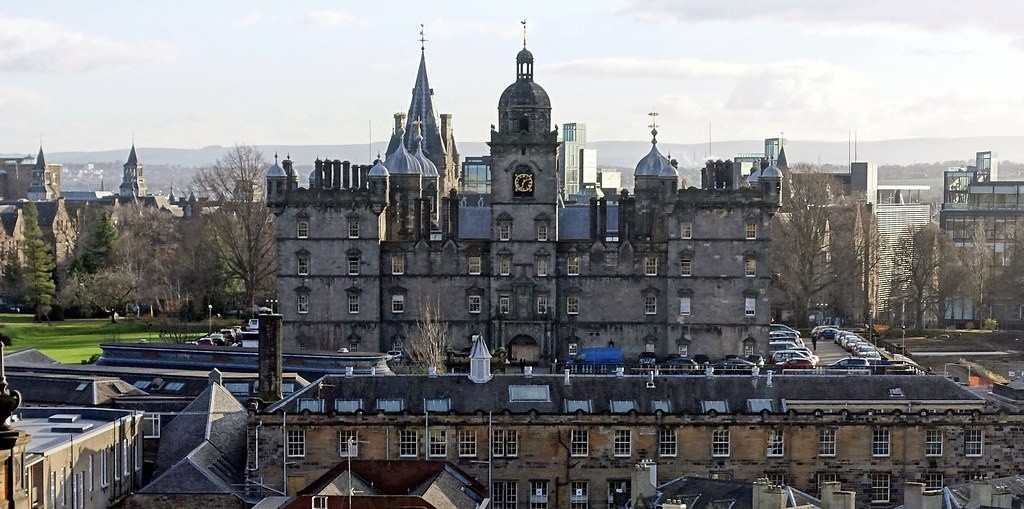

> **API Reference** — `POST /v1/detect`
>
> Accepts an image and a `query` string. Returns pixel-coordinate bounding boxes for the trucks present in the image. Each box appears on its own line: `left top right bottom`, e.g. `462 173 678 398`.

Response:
565 348 624 373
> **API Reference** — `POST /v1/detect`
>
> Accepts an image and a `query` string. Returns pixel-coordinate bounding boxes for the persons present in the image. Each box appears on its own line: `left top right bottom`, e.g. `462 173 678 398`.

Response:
705 365 714 375
812 334 817 351
520 357 526 373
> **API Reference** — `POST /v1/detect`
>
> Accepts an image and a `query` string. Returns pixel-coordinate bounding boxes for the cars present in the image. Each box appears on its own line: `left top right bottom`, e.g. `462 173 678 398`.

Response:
775 358 813 372
712 358 757 375
185 319 259 346
660 359 699 374
811 325 884 360
770 331 819 364
638 352 658 368
769 324 801 337
825 357 870 370
386 351 403 361
748 354 767 368
722 355 739 360
692 355 711 369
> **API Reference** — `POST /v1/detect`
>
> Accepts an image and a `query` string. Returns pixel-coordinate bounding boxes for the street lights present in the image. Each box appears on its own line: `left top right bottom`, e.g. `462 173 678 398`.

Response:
266 300 278 311
209 304 212 334
816 303 828 325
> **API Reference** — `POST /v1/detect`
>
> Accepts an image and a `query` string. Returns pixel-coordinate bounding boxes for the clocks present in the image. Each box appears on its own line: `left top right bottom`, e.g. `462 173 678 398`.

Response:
513 172 533 191
